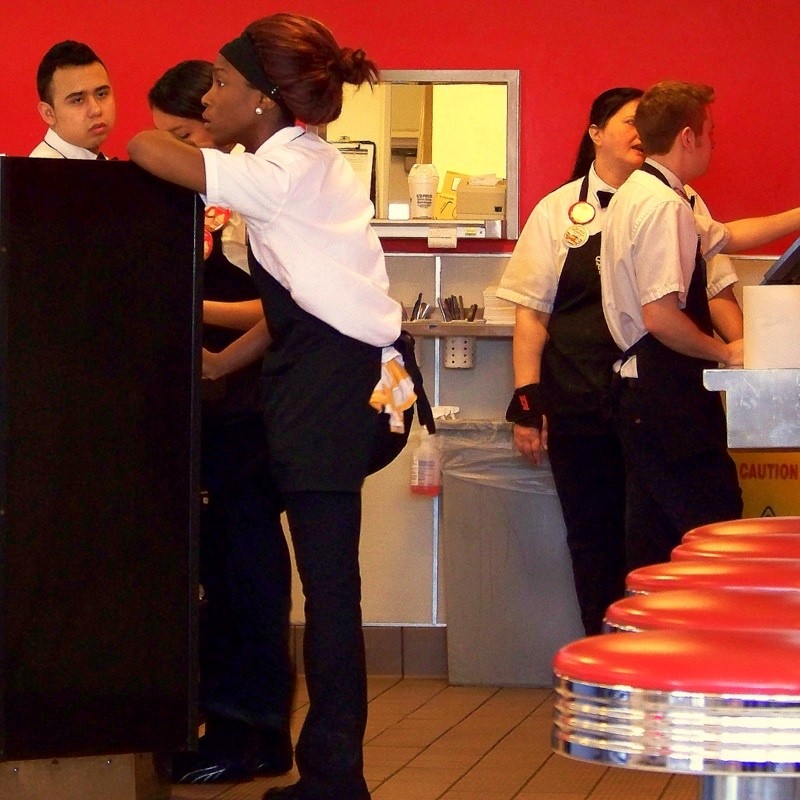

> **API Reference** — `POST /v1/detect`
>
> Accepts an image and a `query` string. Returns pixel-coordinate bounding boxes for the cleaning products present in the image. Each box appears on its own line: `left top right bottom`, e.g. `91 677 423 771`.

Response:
408 406 460 497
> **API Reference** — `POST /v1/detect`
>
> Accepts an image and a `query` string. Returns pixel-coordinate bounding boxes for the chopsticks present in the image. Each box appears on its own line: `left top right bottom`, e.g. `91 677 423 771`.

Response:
399 293 480 368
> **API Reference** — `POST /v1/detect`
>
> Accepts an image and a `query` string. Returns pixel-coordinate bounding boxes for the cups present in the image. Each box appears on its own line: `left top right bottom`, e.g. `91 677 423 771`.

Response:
406 163 439 220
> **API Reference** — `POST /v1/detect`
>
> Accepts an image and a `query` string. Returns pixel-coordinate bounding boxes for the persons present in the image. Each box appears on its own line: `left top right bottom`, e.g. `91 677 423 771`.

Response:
494 83 747 636
124 16 438 799
600 84 746 577
24 38 123 159
146 61 299 727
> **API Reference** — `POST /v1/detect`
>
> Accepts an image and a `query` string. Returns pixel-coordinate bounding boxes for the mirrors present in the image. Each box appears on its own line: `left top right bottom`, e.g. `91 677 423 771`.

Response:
308 71 522 242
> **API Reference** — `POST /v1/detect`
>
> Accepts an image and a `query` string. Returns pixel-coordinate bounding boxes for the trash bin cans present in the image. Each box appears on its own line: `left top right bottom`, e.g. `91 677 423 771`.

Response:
432 414 589 688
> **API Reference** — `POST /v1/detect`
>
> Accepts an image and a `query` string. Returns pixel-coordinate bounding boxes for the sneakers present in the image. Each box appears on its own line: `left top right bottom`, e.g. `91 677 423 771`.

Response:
261 774 371 800
153 735 293 783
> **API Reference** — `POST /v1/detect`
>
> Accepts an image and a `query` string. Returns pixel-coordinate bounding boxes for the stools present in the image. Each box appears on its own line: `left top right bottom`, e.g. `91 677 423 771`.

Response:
550 504 800 800
